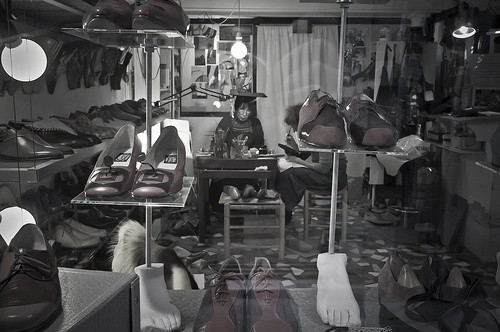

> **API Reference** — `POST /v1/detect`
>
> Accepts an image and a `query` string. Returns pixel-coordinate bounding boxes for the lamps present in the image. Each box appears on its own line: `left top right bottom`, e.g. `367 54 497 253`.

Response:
154 83 268 107
451 0 477 39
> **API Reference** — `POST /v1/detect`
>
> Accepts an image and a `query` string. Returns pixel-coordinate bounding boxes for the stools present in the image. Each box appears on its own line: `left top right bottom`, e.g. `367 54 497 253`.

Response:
218 192 285 262
303 188 348 244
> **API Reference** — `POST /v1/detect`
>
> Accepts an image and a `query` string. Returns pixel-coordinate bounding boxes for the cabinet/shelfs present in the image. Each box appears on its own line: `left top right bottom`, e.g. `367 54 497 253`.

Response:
60 26 195 332
0 0 170 268
291 0 408 327
420 112 500 176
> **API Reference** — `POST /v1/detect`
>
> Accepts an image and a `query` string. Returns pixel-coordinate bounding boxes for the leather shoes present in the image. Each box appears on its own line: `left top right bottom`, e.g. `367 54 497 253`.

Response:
0 222 64 332
131 0 190 38
81 0 132 31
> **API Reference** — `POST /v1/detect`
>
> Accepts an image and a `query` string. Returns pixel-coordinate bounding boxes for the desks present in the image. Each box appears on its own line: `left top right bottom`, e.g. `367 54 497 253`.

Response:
196 156 277 243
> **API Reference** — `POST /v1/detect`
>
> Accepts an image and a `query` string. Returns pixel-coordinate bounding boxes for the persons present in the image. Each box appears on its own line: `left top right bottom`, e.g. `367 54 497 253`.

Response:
210 96 264 211
275 105 347 228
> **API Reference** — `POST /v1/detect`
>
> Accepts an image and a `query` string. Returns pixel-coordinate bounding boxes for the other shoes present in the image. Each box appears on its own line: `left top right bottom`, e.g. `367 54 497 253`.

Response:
191 252 246 332
0 32 169 271
364 211 393 226
344 92 400 150
377 244 500 332
296 88 347 149
246 256 299 332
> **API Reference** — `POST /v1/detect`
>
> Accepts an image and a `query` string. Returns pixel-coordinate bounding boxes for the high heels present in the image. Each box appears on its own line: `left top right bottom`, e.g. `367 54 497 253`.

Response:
131 126 188 200
84 123 144 200
